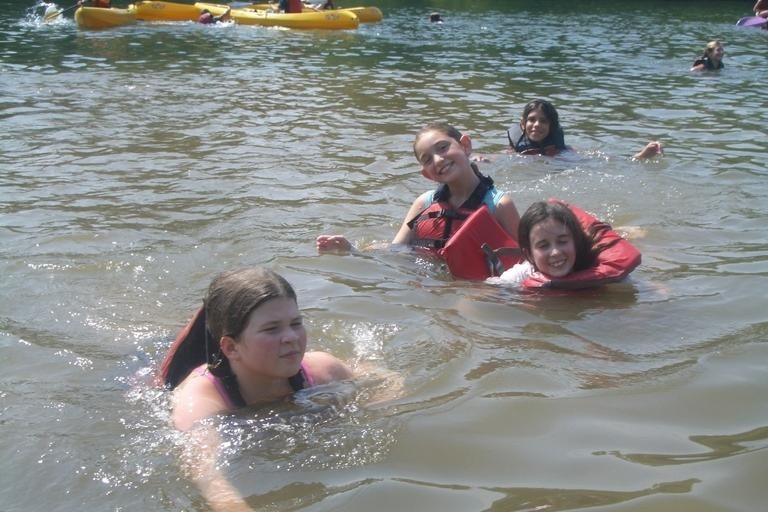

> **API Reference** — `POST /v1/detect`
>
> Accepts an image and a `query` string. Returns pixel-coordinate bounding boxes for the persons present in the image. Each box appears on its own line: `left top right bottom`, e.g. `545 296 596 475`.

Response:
692 39 727 72
753 1 768 21
77 1 112 9
303 0 334 11
466 99 663 165
483 198 624 294
199 4 231 25
265 1 302 14
163 266 361 511
315 120 520 260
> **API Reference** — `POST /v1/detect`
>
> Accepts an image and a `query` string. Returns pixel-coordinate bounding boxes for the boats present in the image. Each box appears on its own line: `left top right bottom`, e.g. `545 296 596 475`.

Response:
735 15 768 30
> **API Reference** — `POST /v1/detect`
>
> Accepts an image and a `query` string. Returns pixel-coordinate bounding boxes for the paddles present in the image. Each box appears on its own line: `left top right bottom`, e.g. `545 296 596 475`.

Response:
44 0 91 20
736 17 767 26
